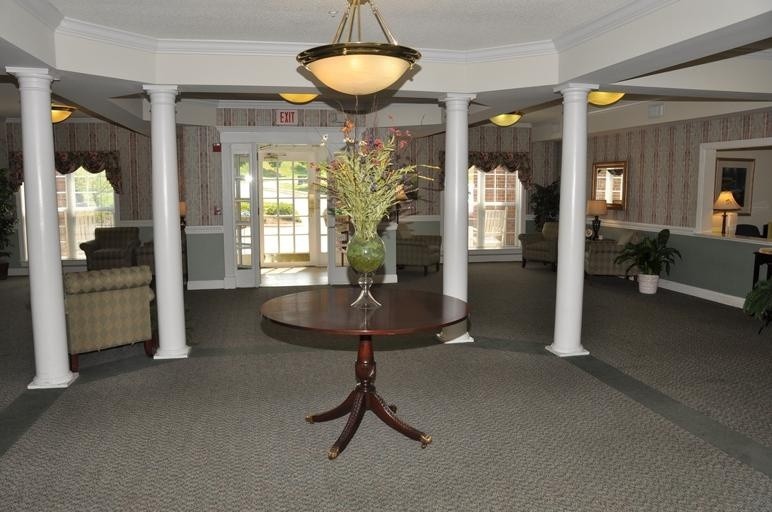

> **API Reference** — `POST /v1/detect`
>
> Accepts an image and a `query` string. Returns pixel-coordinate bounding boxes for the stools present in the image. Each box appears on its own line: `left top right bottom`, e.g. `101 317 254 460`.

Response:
751 250 772 295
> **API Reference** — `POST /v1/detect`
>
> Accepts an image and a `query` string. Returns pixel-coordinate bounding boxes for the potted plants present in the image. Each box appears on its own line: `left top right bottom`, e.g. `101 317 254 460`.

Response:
617 229 684 298
0 169 19 281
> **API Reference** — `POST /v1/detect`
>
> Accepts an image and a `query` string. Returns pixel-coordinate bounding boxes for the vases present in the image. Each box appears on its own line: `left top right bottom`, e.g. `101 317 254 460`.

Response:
343 221 391 312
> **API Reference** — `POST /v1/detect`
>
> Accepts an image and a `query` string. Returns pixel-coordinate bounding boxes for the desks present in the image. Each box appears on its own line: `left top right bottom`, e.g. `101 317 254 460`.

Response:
253 280 476 464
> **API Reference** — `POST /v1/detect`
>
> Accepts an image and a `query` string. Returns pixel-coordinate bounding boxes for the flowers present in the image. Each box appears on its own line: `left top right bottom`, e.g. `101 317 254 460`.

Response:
304 91 442 238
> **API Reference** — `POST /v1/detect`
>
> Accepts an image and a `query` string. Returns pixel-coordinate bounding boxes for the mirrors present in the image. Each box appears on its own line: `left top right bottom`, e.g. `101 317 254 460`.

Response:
591 161 631 213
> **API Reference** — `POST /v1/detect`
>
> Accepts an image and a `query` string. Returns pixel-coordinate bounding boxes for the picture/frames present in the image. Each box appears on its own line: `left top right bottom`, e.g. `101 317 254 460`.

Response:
714 156 757 218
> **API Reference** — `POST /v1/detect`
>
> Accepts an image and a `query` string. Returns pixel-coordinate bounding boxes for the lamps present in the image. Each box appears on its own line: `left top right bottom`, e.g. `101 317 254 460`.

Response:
277 92 320 104
587 92 625 106
713 190 742 235
50 99 78 125
488 111 529 128
179 201 186 227
293 1 424 102
585 199 609 241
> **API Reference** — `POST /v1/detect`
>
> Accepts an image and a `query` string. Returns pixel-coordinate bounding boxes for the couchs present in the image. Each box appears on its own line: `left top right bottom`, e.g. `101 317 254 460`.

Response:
586 228 646 286
516 221 565 274
136 231 190 292
396 226 442 274
62 264 160 372
79 227 141 270
